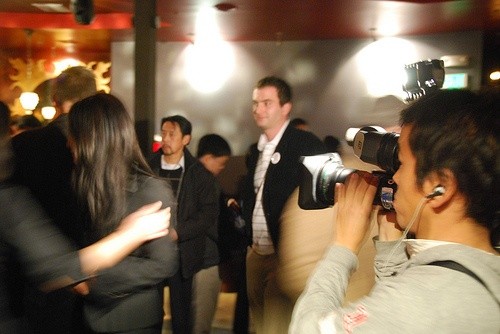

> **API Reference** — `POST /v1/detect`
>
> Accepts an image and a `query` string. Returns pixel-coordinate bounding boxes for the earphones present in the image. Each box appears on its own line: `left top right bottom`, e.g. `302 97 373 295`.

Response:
426 187 445 199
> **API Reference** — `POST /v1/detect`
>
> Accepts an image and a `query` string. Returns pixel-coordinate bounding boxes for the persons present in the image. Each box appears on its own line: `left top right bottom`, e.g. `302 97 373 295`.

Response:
190 118 314 334
245 76 323 334
148 115 219 334
0 66 179 334
287 89 500 334
